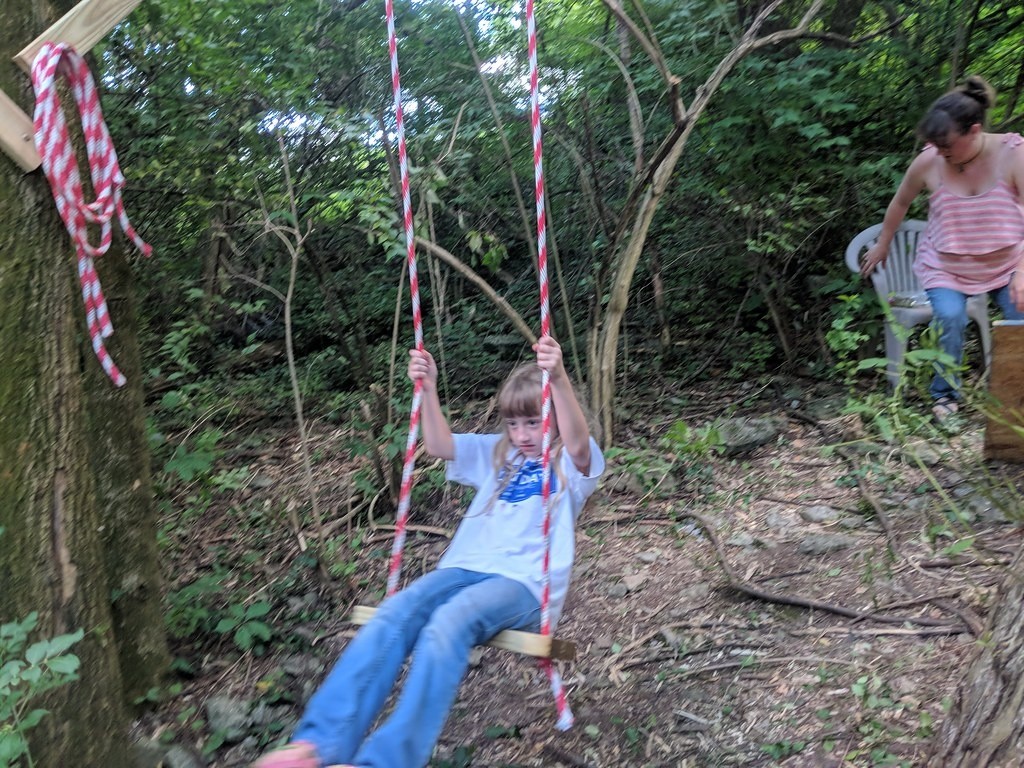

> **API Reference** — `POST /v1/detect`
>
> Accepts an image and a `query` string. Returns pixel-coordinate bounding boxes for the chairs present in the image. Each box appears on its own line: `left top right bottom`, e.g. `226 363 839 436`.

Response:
845 219 995 391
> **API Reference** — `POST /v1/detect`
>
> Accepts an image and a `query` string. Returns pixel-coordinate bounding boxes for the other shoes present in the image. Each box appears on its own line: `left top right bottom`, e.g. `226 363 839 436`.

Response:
929 394 958 425
254 739 323 768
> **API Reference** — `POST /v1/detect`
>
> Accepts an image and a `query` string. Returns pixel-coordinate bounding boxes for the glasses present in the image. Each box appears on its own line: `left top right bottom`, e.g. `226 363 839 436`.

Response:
933 135 964 150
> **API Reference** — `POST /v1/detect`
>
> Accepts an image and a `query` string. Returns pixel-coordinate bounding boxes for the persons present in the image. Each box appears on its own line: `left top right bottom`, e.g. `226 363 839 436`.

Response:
860 76 1024 434
253 338 606 768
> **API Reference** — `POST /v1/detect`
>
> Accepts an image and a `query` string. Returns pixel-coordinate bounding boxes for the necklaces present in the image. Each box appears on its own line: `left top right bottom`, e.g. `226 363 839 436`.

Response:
957 133 986 173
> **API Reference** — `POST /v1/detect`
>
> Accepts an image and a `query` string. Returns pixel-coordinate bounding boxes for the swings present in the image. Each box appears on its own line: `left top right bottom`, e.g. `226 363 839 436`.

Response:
355 0 577 662
